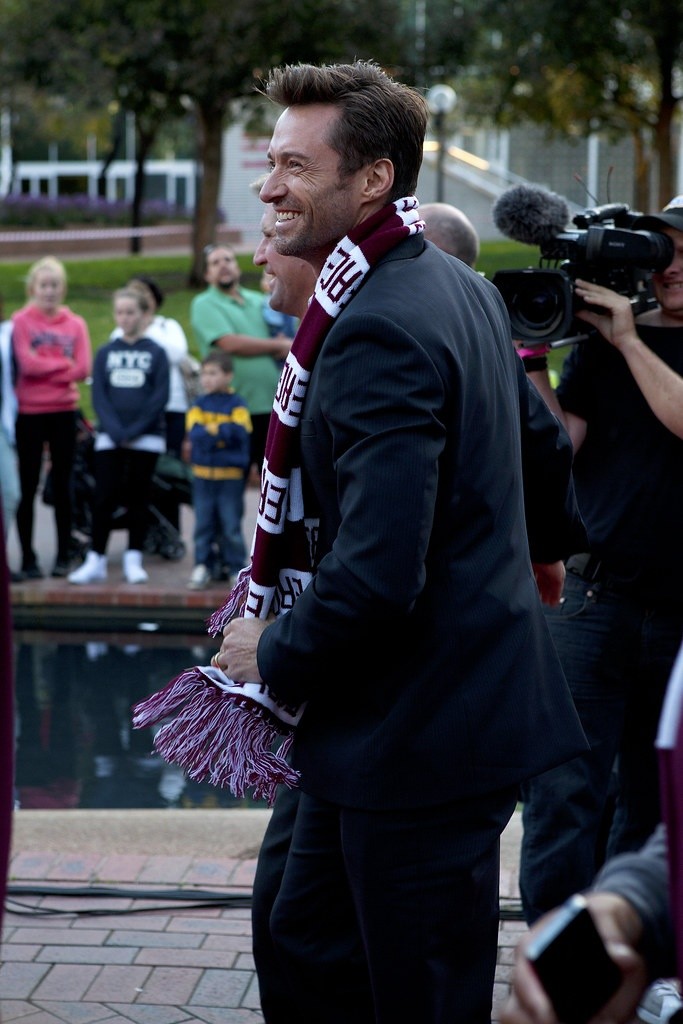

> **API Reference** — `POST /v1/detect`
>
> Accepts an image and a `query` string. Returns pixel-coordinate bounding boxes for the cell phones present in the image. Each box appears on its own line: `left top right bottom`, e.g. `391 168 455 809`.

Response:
521 894 624 1024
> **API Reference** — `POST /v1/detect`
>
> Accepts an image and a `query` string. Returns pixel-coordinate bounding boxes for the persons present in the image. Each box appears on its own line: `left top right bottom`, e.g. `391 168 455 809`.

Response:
11 251 93 580
258 265 303 369
396 200 614 927
184 347 254 593
479 197 682 864
66 286 167 589
211 62 544 1022
505 823 680 1024
249 167 328 320
189 243 294 492
111 272 196 565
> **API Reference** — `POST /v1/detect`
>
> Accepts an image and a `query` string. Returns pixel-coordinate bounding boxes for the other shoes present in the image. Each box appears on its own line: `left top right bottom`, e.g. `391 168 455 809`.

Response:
68 551 108 584
53 556 69 576
122 550 149 584
188 568 211 590
10 574 24 582
22 567 42 578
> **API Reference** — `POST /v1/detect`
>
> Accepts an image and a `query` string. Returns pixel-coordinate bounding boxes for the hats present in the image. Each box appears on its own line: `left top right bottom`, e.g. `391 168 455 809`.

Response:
632 193 682 233
